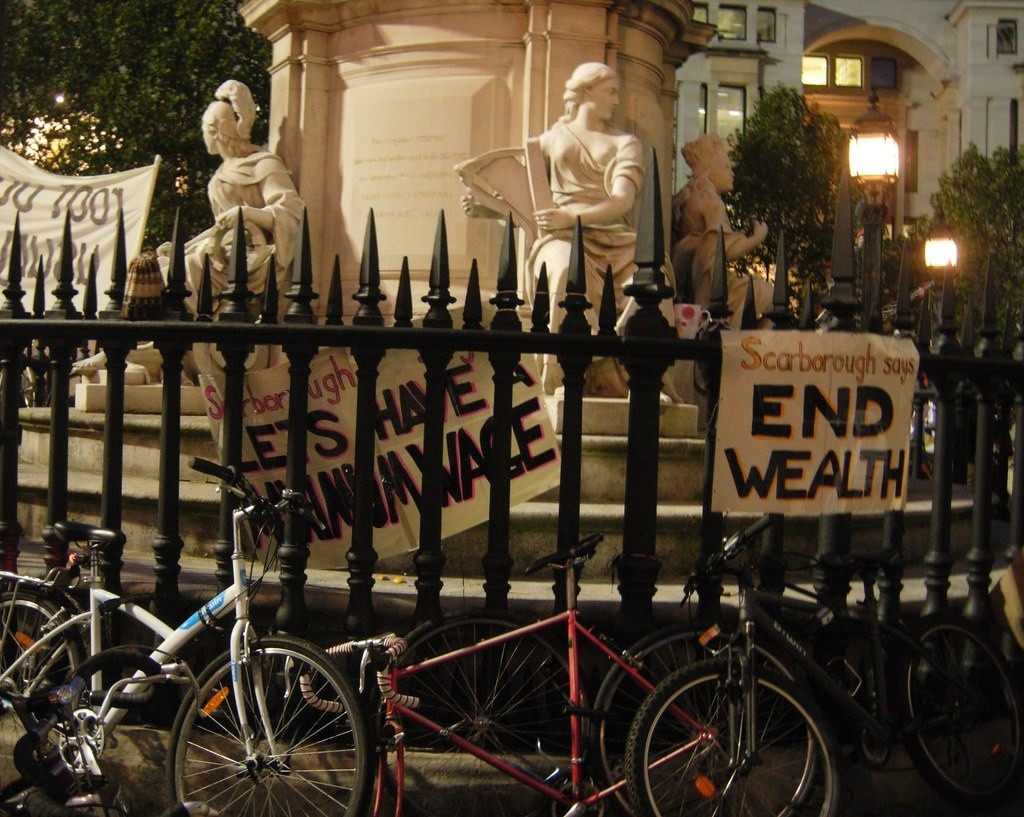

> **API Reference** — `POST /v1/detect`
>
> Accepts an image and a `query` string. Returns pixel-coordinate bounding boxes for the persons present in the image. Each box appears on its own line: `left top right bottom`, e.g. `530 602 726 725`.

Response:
150 79 309 386
669 133 781 333
459 61 686 407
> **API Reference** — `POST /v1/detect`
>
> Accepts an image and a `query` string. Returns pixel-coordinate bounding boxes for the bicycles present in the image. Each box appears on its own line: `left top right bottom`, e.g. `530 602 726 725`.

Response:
2 454 374 815
299 533 821 816
617 510 1022 816
584 511 904 814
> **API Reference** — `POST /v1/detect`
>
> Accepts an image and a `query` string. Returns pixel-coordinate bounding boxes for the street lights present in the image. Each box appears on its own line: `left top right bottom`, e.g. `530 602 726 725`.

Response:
848 85 900 334
924 207 960 352
